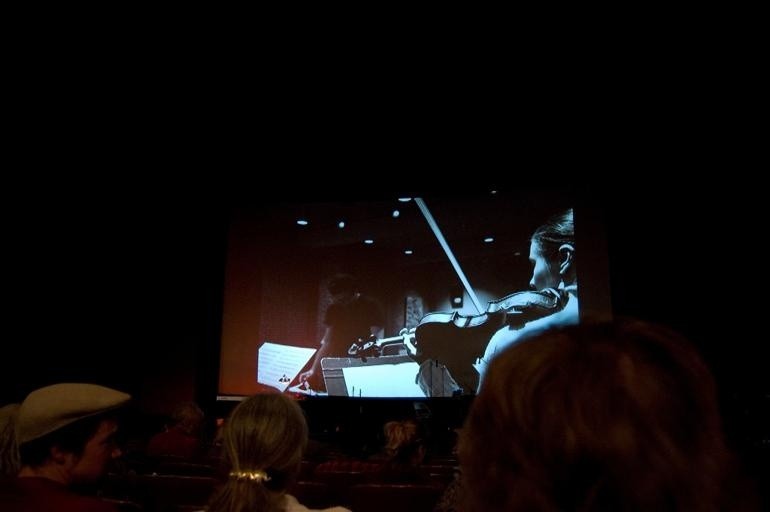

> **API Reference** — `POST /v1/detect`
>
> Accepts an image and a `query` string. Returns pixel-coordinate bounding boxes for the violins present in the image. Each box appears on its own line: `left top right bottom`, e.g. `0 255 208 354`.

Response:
347 290 565 366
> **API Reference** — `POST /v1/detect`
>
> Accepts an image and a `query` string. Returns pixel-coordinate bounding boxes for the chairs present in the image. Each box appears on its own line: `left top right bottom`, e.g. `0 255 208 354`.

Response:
101 455 450 505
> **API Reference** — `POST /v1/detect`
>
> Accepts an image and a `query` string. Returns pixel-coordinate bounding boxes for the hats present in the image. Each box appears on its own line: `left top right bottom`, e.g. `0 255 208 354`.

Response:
15 383 131 448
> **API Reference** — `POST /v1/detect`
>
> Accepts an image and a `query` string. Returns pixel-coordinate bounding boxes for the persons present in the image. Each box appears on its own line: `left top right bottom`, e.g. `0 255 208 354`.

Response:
0 383 308 511
397 206 581 398
296 273 390 396
384 321 732 511
